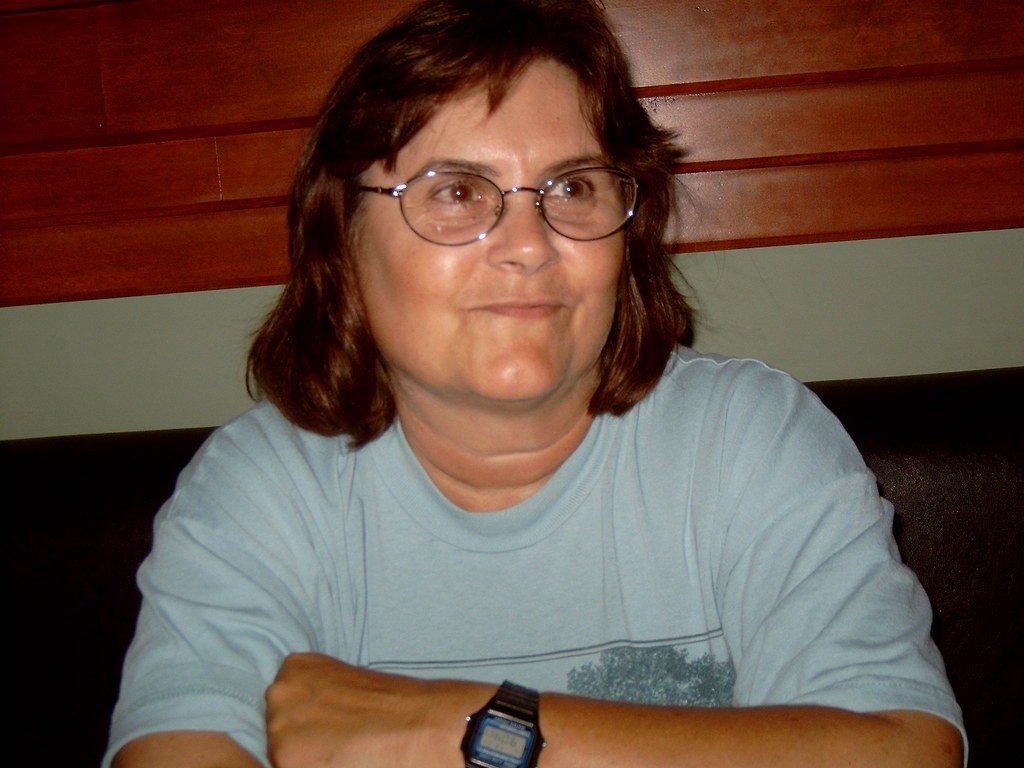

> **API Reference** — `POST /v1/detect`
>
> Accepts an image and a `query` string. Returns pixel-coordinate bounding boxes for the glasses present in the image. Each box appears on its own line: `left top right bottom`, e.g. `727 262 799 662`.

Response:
348 165 648 247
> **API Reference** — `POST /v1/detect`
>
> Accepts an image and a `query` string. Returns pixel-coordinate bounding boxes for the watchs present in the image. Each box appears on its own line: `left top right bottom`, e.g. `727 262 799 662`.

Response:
461 680 546 768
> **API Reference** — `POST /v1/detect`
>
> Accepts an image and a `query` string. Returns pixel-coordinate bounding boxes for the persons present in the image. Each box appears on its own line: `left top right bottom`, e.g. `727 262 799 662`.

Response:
103 0 968 768
